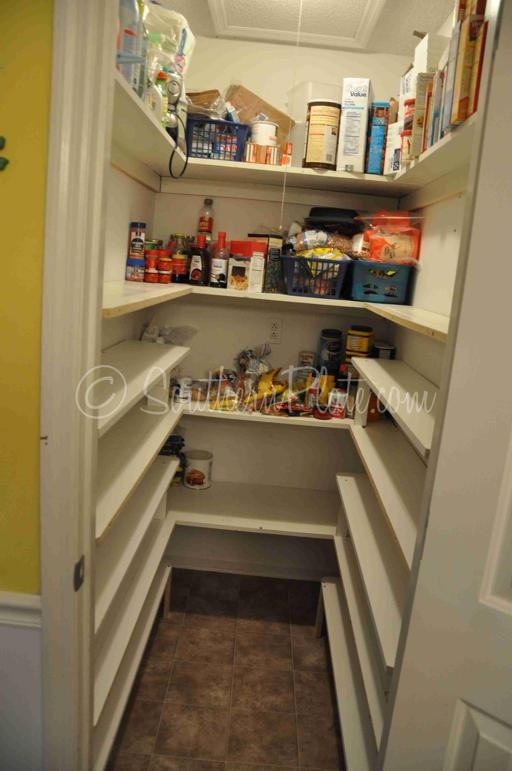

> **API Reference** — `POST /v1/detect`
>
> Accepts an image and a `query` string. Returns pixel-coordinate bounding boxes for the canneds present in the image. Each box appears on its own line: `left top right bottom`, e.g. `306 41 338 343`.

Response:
183 450 213 490
298 325 395 392
126 222 174 284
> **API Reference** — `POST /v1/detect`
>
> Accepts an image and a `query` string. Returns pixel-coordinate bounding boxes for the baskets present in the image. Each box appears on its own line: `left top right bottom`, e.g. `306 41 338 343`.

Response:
185 116 250 163
279 254 350 299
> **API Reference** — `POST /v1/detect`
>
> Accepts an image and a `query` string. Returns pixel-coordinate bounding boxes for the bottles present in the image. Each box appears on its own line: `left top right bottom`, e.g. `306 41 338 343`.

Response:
172 197 230 290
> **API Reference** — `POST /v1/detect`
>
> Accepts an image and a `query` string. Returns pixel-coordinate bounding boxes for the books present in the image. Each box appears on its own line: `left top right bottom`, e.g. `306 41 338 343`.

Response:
407 1 488 159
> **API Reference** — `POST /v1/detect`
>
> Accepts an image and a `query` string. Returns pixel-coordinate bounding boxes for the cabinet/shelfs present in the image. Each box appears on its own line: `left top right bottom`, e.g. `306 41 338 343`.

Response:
309 113 483 771
84 62 191 769
172 155 417 587
